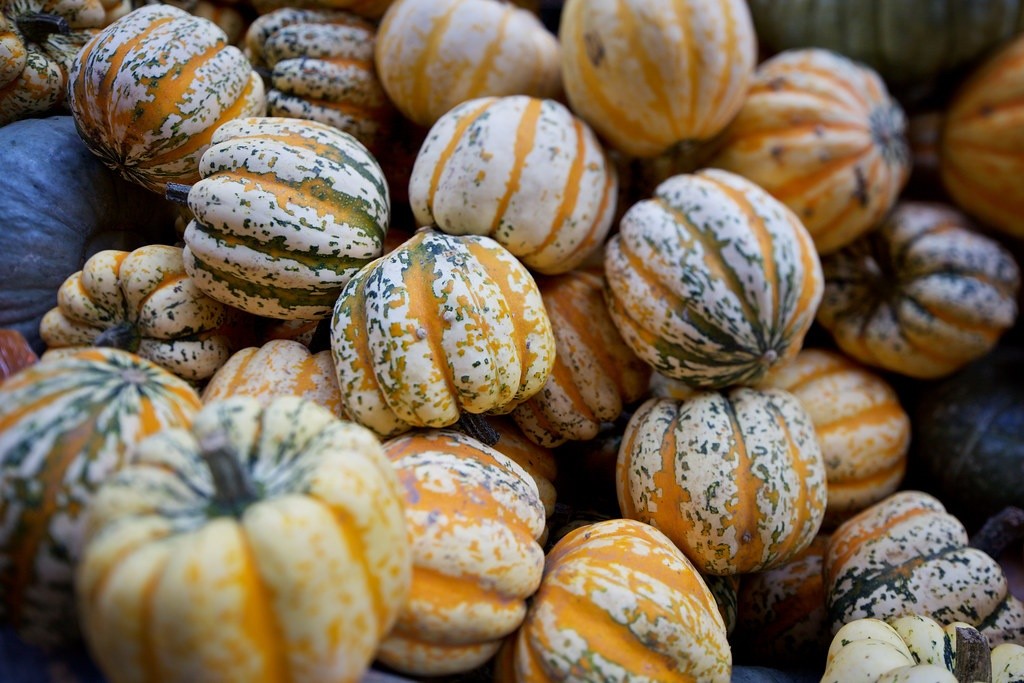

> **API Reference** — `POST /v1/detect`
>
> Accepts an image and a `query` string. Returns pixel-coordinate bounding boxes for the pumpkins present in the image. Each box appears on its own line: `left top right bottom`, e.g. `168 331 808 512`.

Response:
1 0 1024 683
